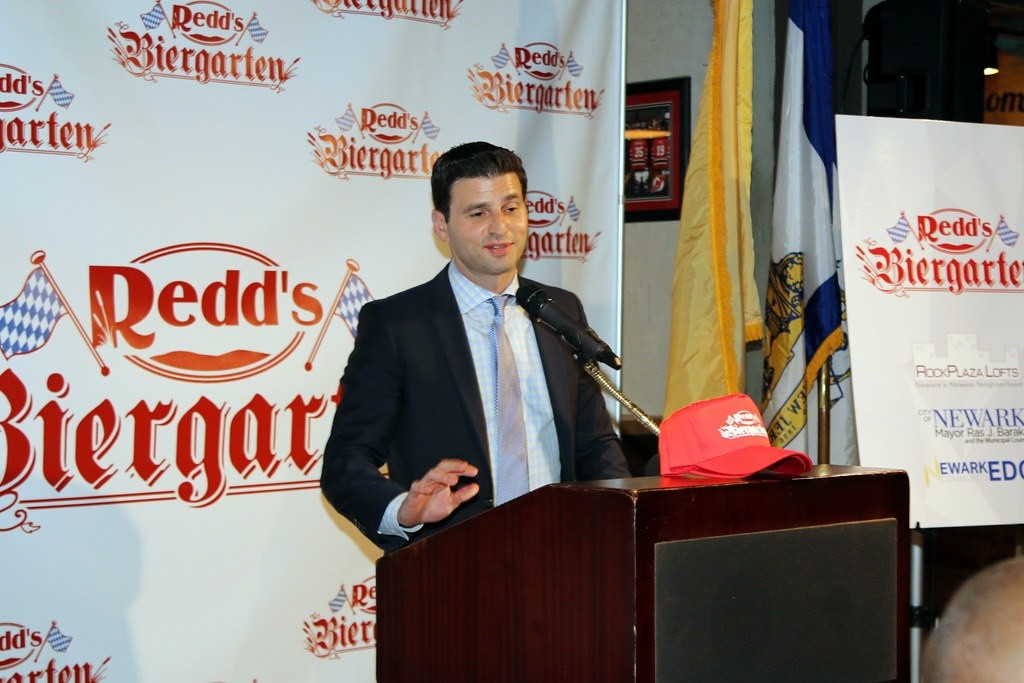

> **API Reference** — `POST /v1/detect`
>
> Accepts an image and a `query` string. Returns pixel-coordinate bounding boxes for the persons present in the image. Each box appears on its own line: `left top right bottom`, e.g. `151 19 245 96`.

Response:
320 142 632 553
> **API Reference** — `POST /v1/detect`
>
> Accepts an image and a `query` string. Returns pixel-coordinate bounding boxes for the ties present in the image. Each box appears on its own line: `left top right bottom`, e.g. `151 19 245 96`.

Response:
488 294 530 506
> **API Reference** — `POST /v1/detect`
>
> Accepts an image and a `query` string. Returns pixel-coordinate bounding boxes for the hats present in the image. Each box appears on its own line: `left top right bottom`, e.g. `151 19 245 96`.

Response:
659 394 814 482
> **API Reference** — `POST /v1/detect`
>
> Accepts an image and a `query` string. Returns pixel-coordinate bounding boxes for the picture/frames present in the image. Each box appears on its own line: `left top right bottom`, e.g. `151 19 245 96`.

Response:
624 76 691 224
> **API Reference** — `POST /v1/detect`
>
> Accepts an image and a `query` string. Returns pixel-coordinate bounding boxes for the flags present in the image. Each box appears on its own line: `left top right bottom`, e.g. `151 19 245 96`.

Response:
663 0 764 419
760 0 860 467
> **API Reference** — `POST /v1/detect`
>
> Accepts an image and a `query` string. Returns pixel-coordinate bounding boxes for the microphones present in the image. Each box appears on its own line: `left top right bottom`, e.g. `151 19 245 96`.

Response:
515 284 623 370
